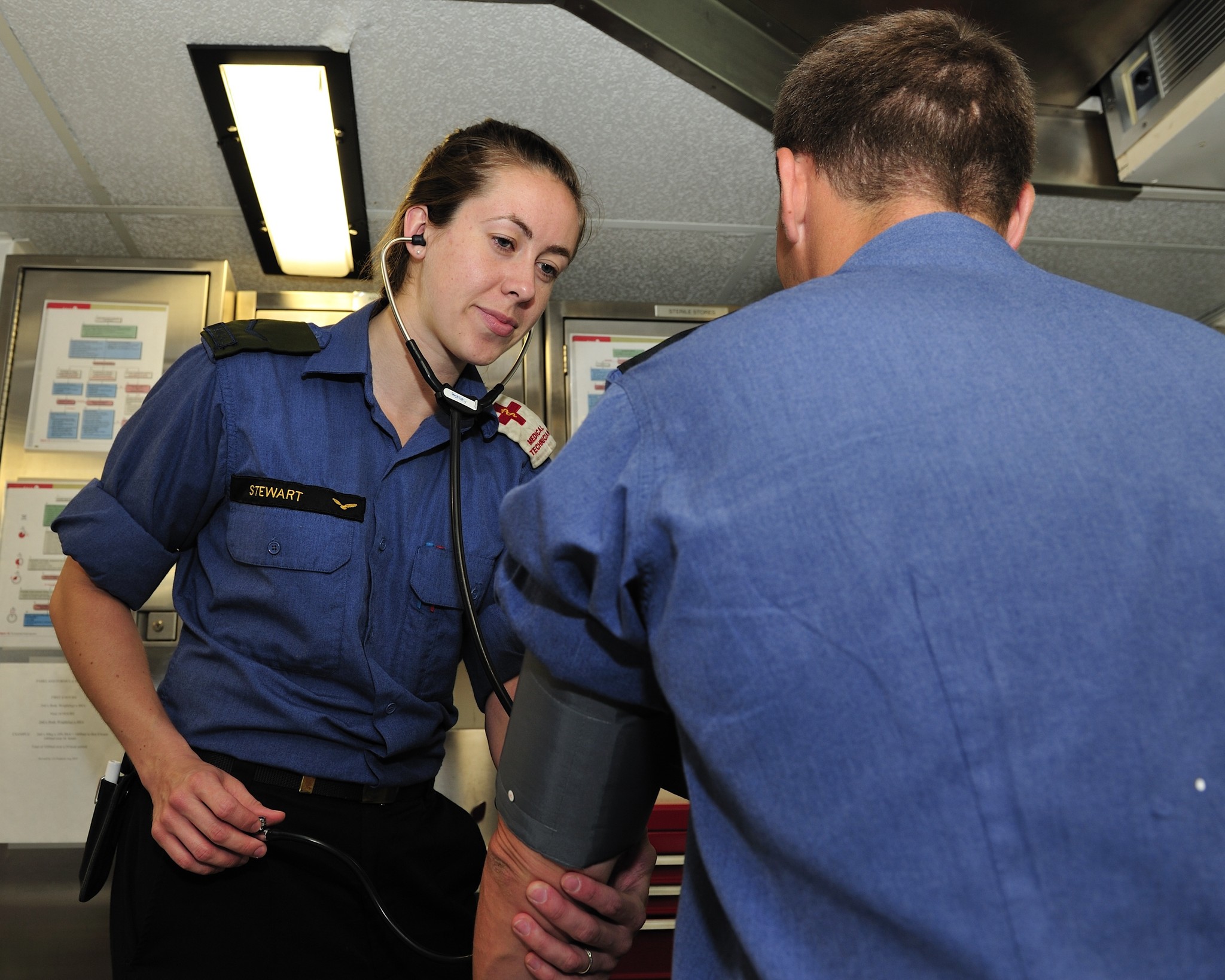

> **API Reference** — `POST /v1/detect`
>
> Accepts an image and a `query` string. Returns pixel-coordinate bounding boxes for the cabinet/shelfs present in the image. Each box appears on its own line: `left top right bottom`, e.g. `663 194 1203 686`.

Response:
0 251 744 979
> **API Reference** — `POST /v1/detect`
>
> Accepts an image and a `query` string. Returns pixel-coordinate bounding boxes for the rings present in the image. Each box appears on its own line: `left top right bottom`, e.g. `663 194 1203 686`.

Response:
576 946 593 973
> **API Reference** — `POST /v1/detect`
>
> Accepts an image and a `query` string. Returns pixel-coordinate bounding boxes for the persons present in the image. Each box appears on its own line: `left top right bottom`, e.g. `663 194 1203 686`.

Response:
31 120 658 980
470 2 1225 977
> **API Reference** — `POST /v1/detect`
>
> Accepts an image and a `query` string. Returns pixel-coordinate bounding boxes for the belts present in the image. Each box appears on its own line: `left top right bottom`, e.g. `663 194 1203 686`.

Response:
190 747 435 807
